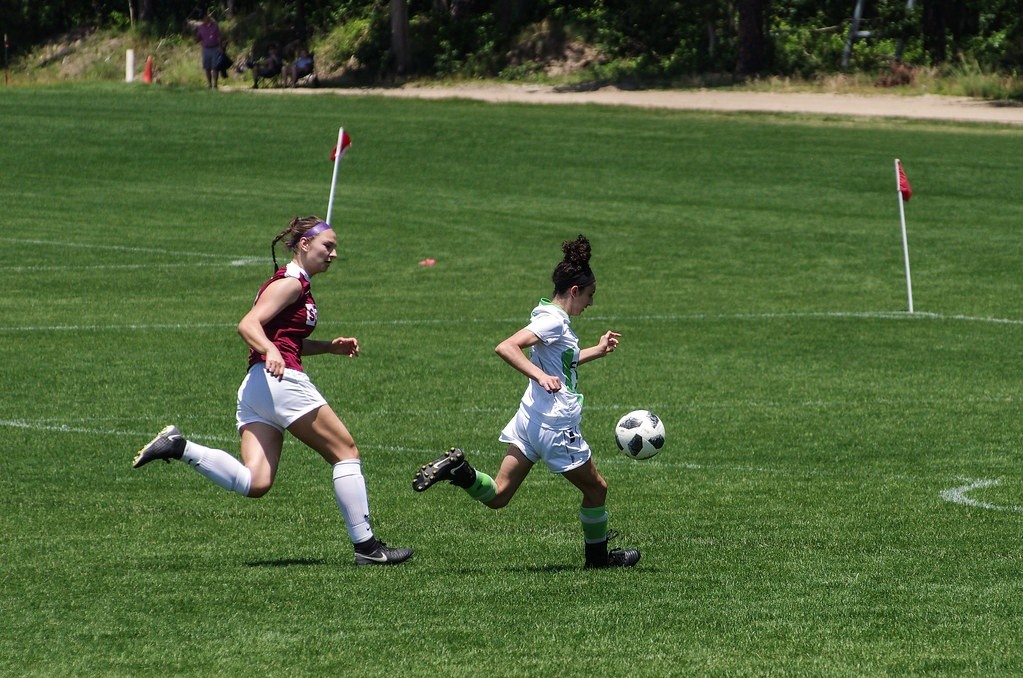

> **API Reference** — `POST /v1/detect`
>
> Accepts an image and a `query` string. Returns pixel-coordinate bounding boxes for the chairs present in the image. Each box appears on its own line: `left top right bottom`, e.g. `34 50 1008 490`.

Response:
257 51 315 89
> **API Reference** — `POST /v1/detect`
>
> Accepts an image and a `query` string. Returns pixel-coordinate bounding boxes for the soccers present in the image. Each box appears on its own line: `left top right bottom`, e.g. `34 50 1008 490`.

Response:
615 409 667 461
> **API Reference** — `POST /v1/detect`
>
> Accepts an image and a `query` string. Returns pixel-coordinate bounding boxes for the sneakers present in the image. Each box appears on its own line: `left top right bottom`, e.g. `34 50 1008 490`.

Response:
132 424 183 470
354 539 415 566
605 548 642 571
411 447 465 493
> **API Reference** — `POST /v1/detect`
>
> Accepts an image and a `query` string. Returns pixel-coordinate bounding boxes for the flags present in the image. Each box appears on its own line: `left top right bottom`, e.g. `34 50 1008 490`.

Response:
330 132 353 161
897 162 913 202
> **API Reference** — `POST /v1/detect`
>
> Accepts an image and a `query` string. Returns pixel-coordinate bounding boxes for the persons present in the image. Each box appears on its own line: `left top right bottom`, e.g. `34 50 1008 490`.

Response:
410 234 641 569
132 217 412 565
248 45 281 90
280 49 312 88
194 13 221 89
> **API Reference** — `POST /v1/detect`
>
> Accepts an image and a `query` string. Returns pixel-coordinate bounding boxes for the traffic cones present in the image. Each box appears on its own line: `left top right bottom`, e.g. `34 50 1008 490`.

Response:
143 56 154 83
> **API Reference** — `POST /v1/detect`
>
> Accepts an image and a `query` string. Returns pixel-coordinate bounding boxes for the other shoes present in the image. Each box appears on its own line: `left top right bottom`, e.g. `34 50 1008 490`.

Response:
282 82 287 88
246 60 254 69
250 83 258 89
289 80 295 87
